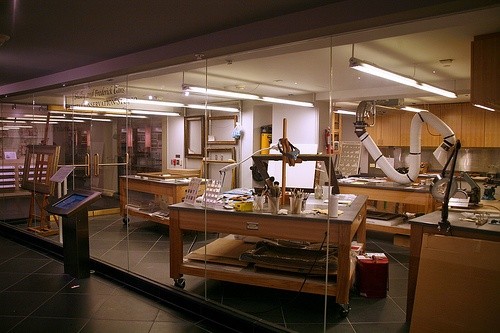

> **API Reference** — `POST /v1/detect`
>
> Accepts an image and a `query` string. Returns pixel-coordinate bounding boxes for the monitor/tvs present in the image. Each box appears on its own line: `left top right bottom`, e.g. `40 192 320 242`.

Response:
54 194 87 210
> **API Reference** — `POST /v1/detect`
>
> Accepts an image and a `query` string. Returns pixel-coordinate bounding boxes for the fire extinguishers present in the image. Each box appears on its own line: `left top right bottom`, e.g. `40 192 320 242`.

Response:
324 127 332 155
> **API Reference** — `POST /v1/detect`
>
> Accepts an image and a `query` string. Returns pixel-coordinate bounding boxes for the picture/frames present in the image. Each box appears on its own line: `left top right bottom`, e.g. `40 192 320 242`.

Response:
203 160 236 189
207 147 236 160
184 115 205 159
207 115 237 145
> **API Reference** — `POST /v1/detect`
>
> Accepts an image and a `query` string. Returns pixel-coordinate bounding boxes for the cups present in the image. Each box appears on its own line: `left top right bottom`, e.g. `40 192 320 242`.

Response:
252 196 263 213
290 197 304 214
323 186 332 202
314 188 322 199
267 196 281 215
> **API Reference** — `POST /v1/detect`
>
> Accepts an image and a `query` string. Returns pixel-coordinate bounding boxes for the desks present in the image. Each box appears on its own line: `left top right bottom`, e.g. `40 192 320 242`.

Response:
405 211 500 333
168 193 369 318
325 177 438 236
437 199 500 213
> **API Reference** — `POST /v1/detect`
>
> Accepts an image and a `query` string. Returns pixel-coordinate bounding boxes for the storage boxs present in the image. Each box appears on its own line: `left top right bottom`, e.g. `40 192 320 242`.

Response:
394 234 411 248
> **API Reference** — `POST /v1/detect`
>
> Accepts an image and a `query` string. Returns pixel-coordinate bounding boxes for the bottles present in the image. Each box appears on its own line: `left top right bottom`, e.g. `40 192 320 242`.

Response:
221 154 223 161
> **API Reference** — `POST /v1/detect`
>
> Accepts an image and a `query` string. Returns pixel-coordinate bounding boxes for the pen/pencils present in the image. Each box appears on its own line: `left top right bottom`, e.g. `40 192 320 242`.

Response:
250 187 267 211
289 188 311 215
323 201 349 204
266 185 282 213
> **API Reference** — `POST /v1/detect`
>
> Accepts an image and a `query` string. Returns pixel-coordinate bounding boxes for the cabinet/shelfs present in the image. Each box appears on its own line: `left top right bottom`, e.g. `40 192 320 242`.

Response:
439 104 485 148
363 104 401 146
485 110 500 148
400 104 439 147
119 175 212 226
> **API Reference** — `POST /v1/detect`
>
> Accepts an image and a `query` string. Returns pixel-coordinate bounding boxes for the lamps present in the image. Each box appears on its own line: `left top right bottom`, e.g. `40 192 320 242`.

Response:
413 79 458 98
259 92 315 108
348 43 416 85
181 71 259 100
65 104 126 112
